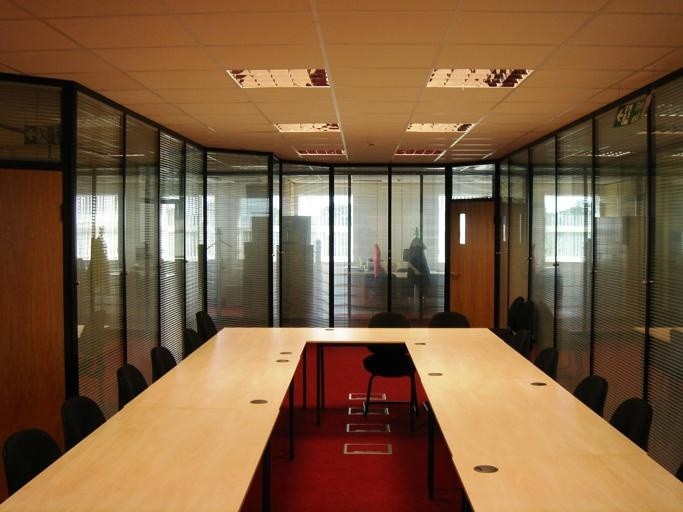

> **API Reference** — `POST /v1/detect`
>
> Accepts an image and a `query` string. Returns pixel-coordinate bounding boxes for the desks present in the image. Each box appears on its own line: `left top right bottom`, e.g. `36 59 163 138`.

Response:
453 454 682 509
393 271 445 310
